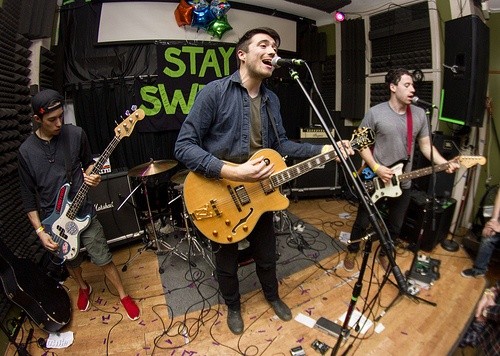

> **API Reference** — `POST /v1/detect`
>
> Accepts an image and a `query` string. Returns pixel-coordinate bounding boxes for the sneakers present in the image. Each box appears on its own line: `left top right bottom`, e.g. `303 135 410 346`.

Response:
462 268 485 278
378 255 391 273
120 294 140 320
77 280 92 311
343 251 357 272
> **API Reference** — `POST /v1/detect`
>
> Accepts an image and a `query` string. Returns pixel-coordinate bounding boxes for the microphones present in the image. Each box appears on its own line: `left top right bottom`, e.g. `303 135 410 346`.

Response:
412 96 438 109
271 56 303 69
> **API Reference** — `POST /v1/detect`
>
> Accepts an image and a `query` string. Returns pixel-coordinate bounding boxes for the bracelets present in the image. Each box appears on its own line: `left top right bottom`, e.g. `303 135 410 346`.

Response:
372 164 379 173
489 218 497 221
35 226 44 234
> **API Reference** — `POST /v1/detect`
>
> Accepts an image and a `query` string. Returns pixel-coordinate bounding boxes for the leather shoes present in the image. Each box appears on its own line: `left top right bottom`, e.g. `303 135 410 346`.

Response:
269 299 292 322
227 310 244 335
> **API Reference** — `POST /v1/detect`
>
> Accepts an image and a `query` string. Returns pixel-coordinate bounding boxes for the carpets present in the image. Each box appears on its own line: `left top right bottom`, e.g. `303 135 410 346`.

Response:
157 211 347 320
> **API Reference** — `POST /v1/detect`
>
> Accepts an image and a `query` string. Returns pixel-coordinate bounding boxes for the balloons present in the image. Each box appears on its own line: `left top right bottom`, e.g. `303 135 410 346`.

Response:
175 0 233 40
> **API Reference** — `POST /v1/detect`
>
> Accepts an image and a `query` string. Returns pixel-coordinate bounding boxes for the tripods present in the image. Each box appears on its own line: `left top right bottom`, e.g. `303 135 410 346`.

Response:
379 108 437 317
122 176 216 273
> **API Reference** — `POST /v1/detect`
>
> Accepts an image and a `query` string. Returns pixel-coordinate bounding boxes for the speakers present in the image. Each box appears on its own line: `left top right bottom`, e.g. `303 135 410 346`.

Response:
85 172 143 248
437 13 490 128
293 138 339 192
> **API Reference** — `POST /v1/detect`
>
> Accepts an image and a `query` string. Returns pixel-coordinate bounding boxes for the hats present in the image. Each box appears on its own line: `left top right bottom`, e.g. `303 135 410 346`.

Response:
31 89 65 119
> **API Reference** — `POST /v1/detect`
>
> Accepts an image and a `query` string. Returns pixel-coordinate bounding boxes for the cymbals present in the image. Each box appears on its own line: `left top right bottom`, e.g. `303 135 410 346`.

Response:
129 159 178 177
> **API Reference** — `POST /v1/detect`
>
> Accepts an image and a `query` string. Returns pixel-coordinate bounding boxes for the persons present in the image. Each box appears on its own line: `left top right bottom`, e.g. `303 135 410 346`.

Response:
174 27 355 335
459 185 500 356
16 90 141 321
343 69 461 274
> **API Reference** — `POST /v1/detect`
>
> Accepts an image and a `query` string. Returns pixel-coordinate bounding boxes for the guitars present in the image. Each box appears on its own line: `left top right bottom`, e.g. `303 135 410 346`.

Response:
38 105 145 260
353 154 486 207
181 127 376 246
